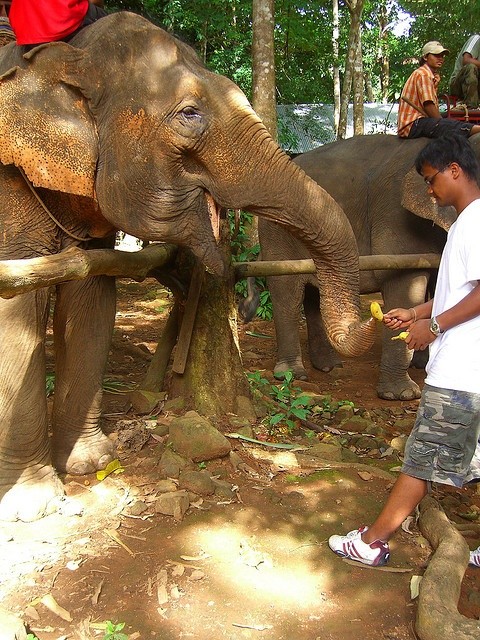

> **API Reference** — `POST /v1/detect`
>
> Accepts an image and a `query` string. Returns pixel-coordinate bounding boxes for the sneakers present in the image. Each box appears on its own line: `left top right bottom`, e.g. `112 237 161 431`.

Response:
328 525 390 568
468 546 480 567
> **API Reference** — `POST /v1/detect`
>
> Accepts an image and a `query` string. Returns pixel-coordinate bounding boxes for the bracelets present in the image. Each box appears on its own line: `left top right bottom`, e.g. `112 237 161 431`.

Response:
408 307 418 323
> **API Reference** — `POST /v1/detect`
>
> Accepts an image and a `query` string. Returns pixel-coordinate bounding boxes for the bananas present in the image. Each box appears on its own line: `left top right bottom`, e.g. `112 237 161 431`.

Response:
391 332 410 341
370 301 384 322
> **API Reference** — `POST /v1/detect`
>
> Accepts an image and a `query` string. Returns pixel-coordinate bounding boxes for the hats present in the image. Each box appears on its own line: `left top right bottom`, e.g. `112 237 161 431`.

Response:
422 40 449 57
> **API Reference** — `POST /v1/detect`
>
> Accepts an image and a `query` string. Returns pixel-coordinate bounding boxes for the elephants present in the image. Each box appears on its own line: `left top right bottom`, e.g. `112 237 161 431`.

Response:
0 9 380 525
256 123 480 403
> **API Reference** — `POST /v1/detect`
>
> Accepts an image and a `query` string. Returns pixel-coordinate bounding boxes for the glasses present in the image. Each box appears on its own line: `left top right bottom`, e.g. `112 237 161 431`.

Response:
423 163 449 185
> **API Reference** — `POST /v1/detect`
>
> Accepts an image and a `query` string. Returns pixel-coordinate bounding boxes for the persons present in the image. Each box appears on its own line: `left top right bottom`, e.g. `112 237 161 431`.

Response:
324 131 480 573
396 40 480 140
448 33 480 110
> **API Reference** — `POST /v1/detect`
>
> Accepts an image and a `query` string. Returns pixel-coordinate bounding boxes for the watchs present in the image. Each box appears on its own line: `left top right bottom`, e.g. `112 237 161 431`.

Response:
429 315 446 338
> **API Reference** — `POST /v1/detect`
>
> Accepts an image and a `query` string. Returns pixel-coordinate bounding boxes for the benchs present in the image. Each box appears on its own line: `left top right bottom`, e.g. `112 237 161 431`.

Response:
441 93 480 124
